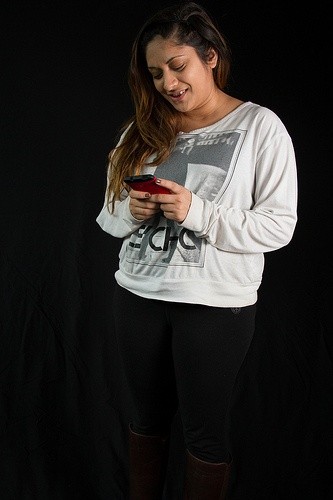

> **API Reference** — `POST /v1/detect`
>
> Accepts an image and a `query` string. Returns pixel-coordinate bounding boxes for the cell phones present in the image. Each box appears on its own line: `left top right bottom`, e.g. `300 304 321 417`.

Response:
123 174 171 194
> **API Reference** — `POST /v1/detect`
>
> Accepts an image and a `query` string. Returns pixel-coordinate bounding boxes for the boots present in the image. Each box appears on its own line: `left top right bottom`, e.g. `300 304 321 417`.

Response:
128 421 171 499
185 447 232 500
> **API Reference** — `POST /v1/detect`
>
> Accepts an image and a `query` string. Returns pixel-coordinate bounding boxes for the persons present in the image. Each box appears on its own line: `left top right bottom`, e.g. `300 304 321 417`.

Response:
95 6 301 500
